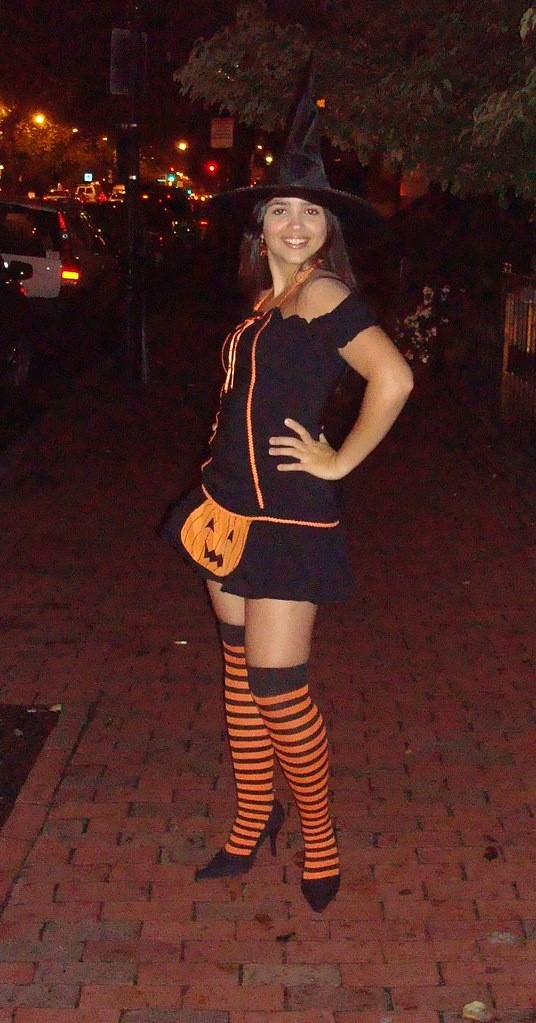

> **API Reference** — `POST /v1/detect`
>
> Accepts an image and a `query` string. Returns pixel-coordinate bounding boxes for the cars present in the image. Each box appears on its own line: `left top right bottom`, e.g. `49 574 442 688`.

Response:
0 180 207 328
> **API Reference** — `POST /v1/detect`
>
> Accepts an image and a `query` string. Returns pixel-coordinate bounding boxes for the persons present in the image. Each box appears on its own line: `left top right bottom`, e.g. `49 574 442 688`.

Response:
166 50 415 914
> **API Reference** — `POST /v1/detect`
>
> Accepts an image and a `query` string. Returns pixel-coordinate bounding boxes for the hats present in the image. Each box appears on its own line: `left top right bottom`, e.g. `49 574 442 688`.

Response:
196 51 385 245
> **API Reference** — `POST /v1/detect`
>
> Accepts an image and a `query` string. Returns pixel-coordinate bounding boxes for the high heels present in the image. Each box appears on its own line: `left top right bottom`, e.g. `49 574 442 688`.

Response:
196 799 284 880
300 817 339 914
